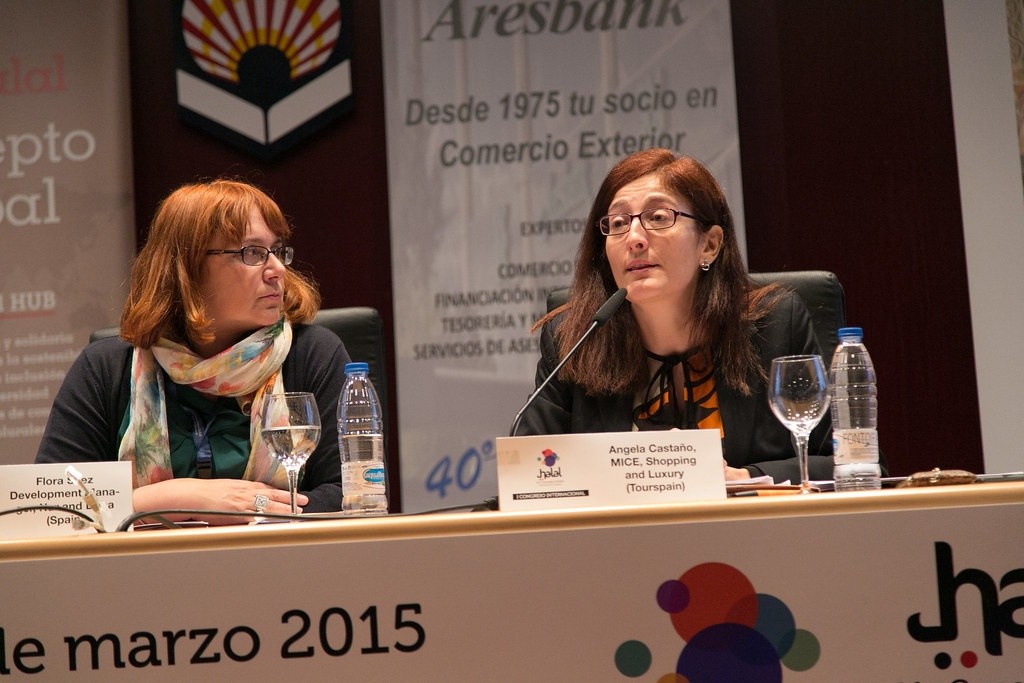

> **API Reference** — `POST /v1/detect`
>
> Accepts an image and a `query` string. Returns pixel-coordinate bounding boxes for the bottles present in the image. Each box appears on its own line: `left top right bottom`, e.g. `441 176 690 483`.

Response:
335 362 389 518
825 327 884 493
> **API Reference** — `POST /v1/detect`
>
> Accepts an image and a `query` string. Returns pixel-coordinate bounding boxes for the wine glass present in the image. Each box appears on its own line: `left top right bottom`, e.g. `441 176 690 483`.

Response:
766 354 832 495
260 391 321 523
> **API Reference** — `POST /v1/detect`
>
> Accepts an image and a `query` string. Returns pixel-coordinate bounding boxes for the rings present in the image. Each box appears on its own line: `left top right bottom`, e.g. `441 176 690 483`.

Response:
254 495 270 511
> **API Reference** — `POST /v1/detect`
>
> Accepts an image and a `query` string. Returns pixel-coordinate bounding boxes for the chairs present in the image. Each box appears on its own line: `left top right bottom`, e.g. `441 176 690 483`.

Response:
537 269 845 502
89 305 391 519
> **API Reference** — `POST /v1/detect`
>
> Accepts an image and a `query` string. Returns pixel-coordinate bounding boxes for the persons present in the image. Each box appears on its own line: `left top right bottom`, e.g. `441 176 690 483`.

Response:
515 148 888 488
35 180 390 525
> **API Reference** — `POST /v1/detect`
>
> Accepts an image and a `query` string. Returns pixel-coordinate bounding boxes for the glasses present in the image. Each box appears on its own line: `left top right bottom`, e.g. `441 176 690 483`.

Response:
206 245 294 266
595 207 697 236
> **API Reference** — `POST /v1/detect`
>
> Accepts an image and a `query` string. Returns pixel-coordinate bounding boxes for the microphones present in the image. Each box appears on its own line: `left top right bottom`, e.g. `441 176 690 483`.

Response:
508 287 627 438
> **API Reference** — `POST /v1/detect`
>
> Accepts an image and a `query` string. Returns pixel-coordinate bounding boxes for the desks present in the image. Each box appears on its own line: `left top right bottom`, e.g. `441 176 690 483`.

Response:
0 476 1024 683
